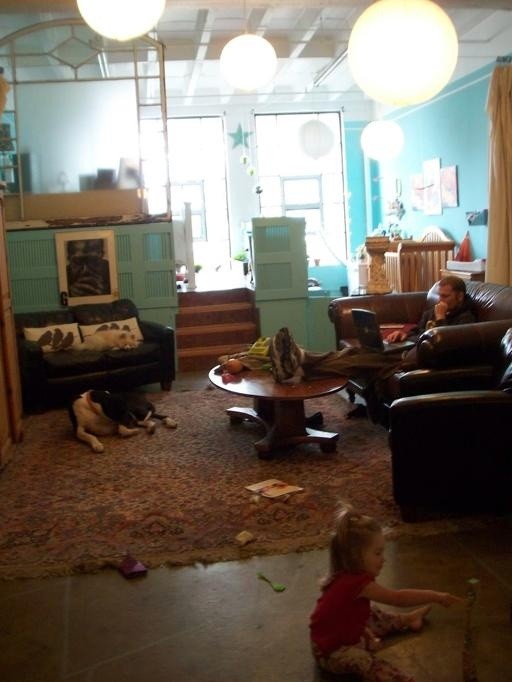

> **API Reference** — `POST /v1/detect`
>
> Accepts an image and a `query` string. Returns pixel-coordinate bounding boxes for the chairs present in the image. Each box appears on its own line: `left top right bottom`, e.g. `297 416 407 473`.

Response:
389 327 512 522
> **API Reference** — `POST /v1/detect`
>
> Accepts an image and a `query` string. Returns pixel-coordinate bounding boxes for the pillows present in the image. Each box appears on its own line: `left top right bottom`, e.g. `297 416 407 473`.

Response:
79 316 144 349
23 322 81 352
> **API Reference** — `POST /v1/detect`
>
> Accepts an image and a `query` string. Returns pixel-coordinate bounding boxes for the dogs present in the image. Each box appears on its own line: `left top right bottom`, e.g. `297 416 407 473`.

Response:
68 388 178 454
66 329 140 353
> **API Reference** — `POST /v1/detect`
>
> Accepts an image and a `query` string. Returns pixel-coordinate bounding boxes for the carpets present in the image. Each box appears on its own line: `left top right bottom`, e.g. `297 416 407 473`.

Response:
0 388 460 580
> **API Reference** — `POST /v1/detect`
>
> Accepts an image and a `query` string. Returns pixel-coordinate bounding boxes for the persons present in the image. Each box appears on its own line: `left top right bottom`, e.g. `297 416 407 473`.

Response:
308 500 462 682
268 275 478 385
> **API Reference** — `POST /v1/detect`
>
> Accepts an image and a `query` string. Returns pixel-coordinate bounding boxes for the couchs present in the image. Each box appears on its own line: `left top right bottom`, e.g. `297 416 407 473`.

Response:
328 279 512 430
14 298 176 416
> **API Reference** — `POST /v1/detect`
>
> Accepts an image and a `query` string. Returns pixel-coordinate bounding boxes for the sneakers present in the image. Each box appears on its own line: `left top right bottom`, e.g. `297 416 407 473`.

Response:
269 326 306 386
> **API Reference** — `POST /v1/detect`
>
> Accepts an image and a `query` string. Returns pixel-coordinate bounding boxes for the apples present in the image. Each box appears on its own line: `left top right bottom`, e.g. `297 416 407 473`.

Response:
227 359 241 374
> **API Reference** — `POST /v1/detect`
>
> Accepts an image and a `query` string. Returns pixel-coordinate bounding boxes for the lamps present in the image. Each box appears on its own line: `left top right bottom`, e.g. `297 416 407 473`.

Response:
220 1 277 92
78 0 166 41
347 0 459 104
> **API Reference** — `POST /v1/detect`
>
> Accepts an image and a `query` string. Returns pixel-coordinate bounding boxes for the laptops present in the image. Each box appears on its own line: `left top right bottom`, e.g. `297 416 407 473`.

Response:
351 308 415 355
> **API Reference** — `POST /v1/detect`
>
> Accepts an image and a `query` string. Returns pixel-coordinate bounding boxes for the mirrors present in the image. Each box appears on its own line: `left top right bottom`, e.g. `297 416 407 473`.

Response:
54 230 119 306
